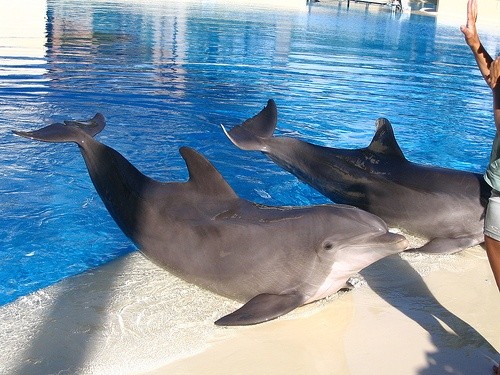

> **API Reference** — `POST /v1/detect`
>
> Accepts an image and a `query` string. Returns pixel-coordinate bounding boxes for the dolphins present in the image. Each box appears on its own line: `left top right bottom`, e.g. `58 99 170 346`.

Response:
12 113 408 326
221 99 492 255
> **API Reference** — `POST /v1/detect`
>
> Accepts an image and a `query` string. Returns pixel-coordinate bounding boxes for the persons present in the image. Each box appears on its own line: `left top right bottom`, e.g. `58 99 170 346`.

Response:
460 0 500 375
